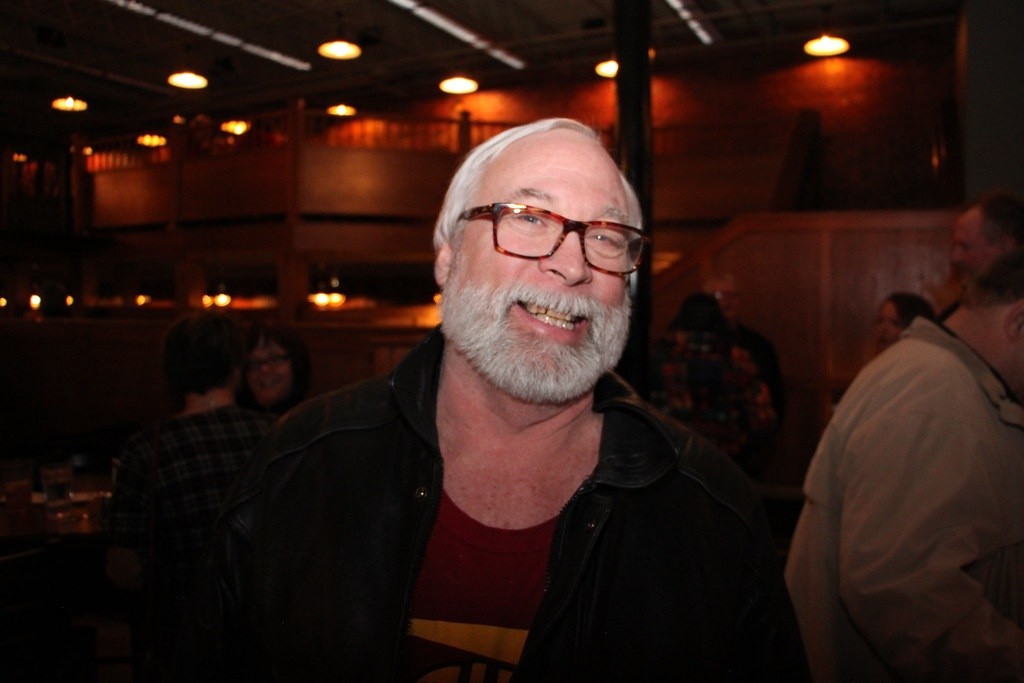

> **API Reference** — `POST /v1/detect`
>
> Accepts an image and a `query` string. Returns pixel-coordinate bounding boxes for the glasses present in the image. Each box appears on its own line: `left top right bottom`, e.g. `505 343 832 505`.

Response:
249 354 290 369
455 202 650 274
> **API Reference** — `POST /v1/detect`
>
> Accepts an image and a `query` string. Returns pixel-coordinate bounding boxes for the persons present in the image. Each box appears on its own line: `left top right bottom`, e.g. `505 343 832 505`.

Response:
104 311 268 683
783 192 1024 683
236 322 312 416
648 289 784 454
212 116 767 683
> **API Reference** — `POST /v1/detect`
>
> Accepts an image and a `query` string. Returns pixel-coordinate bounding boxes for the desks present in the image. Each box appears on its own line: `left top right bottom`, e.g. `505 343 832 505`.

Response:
0 457 122 683
